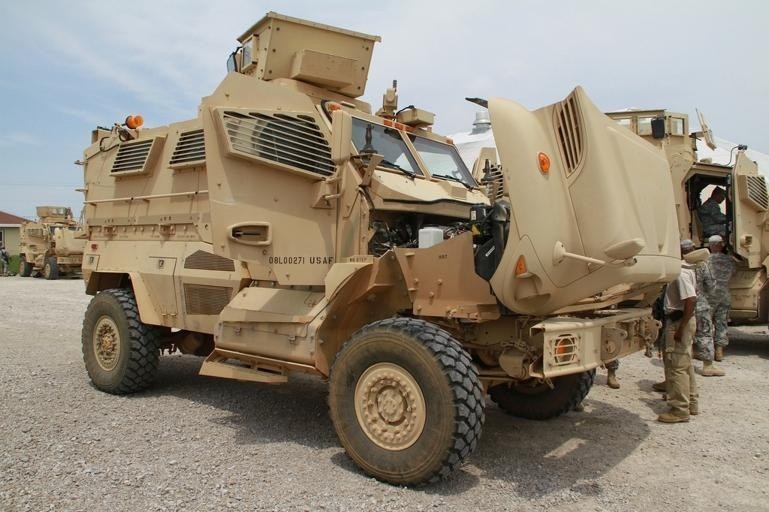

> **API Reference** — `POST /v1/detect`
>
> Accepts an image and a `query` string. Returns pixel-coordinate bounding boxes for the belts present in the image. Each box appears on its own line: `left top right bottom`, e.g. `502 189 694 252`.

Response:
664 312 685 321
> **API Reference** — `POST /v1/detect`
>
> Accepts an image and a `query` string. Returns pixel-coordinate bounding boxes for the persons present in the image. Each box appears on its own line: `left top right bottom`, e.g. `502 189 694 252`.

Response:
657 260 697 423
573 358 621 413
644 186 739 377
0 245 9 277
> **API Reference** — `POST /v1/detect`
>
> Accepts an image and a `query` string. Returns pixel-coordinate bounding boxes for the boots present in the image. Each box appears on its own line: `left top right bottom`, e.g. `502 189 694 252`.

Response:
606 369 620 389
702 360 726 376
715 345 725 360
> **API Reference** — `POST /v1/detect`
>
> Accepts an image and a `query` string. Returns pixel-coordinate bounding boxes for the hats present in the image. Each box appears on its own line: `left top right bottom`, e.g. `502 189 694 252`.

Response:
714 186 726 196
681 239 695 250
708 235 723 244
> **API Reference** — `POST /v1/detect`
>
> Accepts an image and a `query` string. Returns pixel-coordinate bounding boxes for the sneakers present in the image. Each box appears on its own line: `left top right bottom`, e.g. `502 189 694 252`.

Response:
652 409 699 422
653 381 668 400
